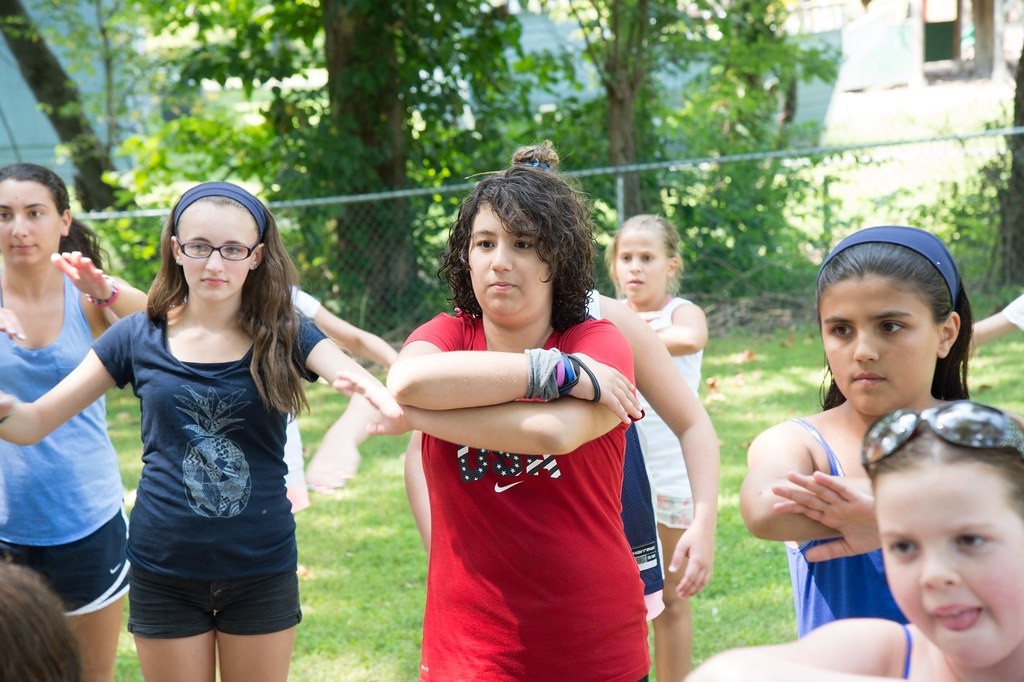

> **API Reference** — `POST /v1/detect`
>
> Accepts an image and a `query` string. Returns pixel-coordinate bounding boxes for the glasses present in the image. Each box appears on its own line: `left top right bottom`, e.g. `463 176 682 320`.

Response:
175 235 261 261
860 400 1023 479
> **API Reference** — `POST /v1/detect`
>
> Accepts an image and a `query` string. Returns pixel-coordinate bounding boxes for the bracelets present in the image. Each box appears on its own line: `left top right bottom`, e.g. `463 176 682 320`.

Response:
85 276 122 308
568 351 600 404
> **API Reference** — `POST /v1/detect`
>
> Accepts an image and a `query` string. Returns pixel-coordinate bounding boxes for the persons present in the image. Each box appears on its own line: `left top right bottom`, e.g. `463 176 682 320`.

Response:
0 163 158 682
968 293 1024 350
1 183 406 682
742 224 975 640
0 560 80 682
682 397 1023 681
509 139 724 627
333 159 644 679
268 272 401 510
602 215 706 682
